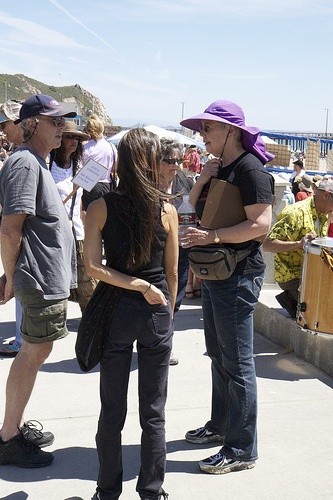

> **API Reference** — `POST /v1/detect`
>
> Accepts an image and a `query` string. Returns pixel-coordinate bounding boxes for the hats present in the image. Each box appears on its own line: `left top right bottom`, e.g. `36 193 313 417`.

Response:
176 100 279 164
0 101 24 123
310 173 323 185
186 144 197 149
15 94 77 125
62 120 92 142
296 174 312 193
317 175 333 193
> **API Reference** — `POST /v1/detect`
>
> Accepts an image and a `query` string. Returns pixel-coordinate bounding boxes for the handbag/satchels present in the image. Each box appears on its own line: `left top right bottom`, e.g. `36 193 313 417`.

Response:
112 178 118 191
71 265 120 374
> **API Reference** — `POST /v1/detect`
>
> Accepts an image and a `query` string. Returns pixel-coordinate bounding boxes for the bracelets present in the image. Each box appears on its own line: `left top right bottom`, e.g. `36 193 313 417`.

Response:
142 283 152 295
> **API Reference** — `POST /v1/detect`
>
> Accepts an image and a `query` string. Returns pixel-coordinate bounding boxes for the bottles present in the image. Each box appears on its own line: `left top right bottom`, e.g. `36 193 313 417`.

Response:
177 195 197 246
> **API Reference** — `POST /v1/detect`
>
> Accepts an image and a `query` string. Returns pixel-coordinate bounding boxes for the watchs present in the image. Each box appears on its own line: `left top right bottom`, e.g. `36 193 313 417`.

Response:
213 229 221 245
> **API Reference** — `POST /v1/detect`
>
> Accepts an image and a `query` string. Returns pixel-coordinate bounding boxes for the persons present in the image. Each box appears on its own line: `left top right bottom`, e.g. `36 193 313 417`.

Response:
182 141 210 174
0 95 78 470
286 149 333 203
176 99 277 476
0 100 27 357
263 179 333 319
81 128 179 500
79 114 118 213
43 120 102 317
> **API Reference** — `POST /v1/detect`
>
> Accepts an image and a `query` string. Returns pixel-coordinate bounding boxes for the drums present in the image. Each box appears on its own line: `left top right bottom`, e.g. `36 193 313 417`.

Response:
295 237 333 335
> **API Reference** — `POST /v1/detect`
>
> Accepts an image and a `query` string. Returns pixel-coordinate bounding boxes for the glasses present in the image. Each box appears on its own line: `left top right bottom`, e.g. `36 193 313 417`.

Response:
161 156 184 165
36 116 67 127
0 121 13 130
198 123 223 133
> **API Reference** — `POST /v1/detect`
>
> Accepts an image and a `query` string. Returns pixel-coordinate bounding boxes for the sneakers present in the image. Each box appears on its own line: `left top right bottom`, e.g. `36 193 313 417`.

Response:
1 430 55 469
168 353 179 366
185 421 232 446
198 449 261 475
18 419 55 448
140 485 168 499
274 291 297 318
90 490 105 500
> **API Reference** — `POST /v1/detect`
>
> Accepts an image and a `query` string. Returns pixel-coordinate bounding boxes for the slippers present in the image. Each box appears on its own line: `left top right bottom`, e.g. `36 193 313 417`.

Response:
0 339 24 358
